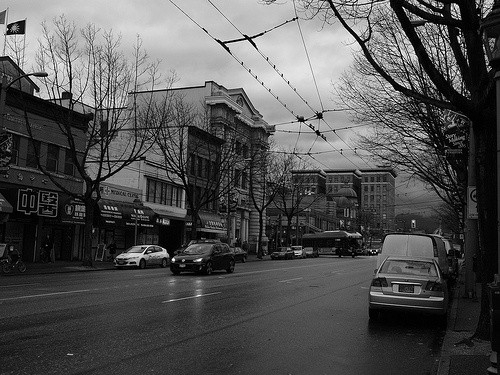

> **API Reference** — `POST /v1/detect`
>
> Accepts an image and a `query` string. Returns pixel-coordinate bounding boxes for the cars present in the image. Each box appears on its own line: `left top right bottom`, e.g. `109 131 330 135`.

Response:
367 254 449 321
270 246 295 260
113 244 170 270
304 246 319 258
230 246 248 263
290 245 307 259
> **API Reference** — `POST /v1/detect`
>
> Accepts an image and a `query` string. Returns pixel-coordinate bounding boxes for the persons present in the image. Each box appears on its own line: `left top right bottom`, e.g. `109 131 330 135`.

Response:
235 237 247 251
220 235 231 244
45 235 53 264
105 241 117 261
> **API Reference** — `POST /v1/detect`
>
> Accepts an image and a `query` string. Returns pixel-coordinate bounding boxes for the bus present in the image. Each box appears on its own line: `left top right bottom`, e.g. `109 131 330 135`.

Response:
300 229 366 256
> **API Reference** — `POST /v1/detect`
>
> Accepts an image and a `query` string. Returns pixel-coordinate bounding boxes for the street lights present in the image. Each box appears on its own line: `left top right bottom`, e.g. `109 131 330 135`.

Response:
227 157 252 245
296 186 316 245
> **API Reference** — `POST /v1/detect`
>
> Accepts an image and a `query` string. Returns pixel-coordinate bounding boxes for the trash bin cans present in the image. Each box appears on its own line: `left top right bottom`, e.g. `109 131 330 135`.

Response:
488 283 500 352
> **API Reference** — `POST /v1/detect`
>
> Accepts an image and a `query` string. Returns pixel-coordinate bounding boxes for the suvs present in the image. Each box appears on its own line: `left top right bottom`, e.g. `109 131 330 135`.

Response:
169 238 236 276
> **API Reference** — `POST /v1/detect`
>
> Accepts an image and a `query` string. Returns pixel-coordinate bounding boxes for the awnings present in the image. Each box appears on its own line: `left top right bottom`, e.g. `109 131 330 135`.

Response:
196 211 224 233
153 208 192 222
0 193 13 213
92 198 157 228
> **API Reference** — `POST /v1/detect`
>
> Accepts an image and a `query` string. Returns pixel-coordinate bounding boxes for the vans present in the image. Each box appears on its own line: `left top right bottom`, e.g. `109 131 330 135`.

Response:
373 233 462 286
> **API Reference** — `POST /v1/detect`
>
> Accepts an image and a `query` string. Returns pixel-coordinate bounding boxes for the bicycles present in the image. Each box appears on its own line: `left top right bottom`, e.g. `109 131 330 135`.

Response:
0 246 28 274
